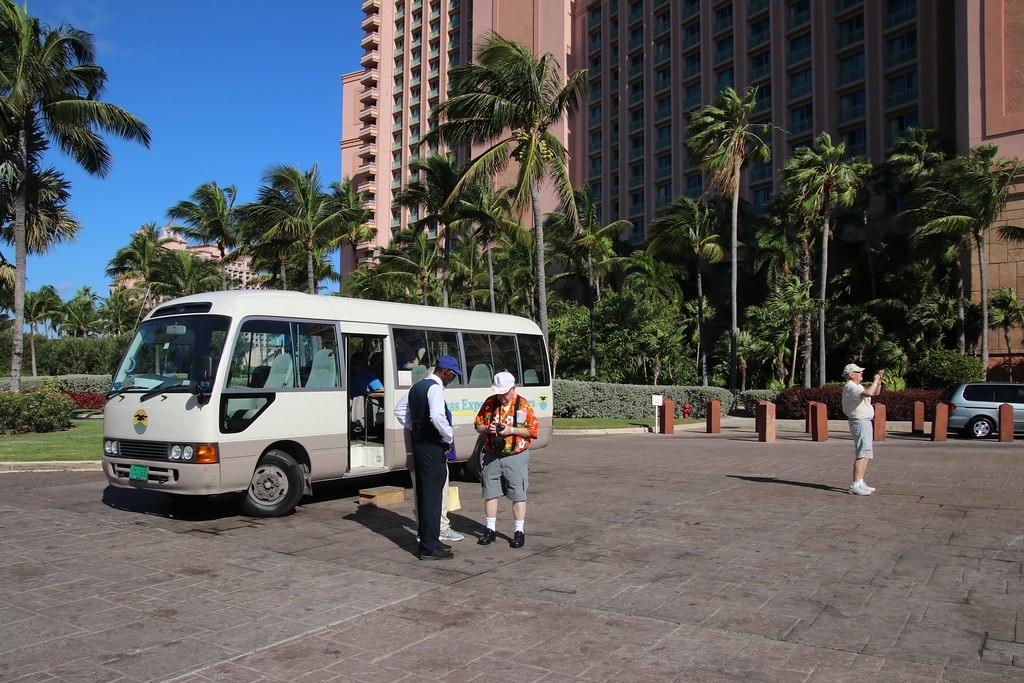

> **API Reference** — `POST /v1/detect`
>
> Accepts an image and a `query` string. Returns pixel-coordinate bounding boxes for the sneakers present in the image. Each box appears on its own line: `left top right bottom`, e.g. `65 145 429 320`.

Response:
420 540 454 561
510 530 525 548
438 528 464 541
848 483 876 496
477 528 496 545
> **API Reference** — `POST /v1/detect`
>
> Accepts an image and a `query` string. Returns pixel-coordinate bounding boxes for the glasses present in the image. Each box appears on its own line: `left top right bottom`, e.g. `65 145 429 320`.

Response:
449 370 458 377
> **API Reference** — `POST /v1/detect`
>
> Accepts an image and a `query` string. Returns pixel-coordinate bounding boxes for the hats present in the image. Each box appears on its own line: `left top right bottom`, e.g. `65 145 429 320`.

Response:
435 354 464 376
490 372 515 395
842 364 866 377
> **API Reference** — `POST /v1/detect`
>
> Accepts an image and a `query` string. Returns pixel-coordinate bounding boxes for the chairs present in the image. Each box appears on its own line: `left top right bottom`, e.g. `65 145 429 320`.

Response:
304 349 336 388
468 363 493 388
411 365 460 386
524 368 547 385
230 353 294 419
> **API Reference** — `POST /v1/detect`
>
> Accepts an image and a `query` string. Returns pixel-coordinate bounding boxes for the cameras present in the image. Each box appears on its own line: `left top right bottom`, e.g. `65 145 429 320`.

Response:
492 423 505 432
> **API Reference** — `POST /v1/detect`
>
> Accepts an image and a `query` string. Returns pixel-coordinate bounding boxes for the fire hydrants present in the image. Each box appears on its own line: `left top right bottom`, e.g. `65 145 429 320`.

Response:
683 404 690 418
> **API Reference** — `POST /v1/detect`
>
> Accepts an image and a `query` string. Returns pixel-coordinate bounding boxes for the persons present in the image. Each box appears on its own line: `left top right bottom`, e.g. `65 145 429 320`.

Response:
842 363 885 497
347 335 387 437
473 372 539 548
394 355 465 560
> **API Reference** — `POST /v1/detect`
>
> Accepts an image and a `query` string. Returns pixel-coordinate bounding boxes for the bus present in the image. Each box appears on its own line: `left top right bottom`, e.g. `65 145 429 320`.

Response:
102 290 553 517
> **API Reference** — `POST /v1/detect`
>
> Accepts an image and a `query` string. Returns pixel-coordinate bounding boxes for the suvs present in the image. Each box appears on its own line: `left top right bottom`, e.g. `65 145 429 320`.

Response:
948 381 1024 439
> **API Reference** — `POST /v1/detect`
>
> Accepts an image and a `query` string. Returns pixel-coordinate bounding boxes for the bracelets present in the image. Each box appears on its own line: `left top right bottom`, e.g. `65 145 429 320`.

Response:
510 427 513 434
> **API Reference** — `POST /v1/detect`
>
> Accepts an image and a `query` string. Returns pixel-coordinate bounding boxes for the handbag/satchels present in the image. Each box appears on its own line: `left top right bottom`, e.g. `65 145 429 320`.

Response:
446 486 461 512
